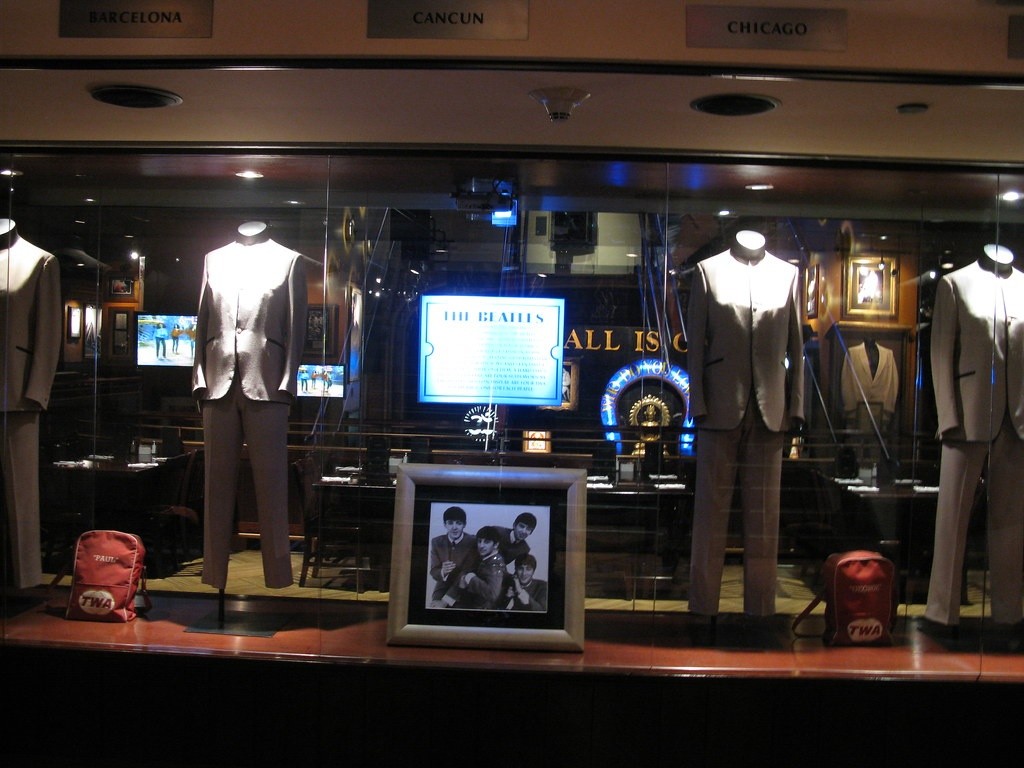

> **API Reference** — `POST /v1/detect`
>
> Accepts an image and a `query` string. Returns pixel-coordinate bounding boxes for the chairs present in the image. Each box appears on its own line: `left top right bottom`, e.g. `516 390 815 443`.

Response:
38 433 204 579
291 451 368 593
802 462 987 603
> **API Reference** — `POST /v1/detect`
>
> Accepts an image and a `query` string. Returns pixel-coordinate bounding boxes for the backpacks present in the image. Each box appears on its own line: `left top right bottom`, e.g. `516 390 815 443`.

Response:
791 550 897 646
43 531 152 622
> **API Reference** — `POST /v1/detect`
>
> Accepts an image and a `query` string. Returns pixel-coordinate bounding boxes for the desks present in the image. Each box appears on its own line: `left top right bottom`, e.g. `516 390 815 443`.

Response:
815 472 969 604
586 472 696 601
315 462 395 595
52 444 204 579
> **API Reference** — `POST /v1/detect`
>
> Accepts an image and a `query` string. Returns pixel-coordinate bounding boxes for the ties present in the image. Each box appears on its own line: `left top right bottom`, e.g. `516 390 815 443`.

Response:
513 541 516 545
450 541 455 550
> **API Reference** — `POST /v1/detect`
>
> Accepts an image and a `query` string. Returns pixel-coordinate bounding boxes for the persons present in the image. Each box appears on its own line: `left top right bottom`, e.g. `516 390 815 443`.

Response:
429 506 479 608
640 405 660 427
300 370 309 393
171 323 185 354
686 230 806 627
155 323 168 360
186 324 196 359
840 339 899 460
0 216 58 592
322 371 330 391
493 512 537 575
498 553 547 611
448 526 504 609
924 243 1024 626
190 221 308 591
311 370 319 389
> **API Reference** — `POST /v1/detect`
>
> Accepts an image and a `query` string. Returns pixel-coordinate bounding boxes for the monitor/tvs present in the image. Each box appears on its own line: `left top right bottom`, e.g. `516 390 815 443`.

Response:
296 363 348 400
133 310 198 369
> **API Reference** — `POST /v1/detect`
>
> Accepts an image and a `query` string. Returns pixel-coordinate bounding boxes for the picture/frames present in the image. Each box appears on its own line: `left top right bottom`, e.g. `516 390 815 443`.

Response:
522 430 552 453
386 463 588 653
304 304 340 357
840 249 899 321
536 357 578 412
805 264 819 319
67 269 135 359
